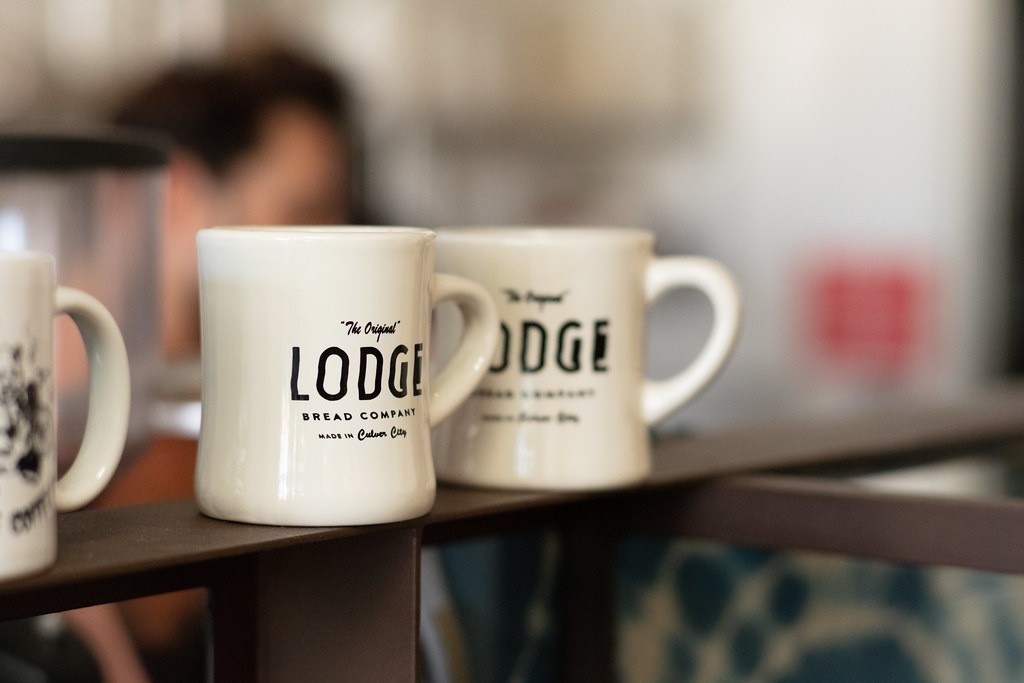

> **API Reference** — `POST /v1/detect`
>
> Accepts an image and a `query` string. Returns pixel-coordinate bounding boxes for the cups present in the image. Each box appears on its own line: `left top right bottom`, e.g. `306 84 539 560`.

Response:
432 221 741 494
0 247 131 583
194 225 500 527
